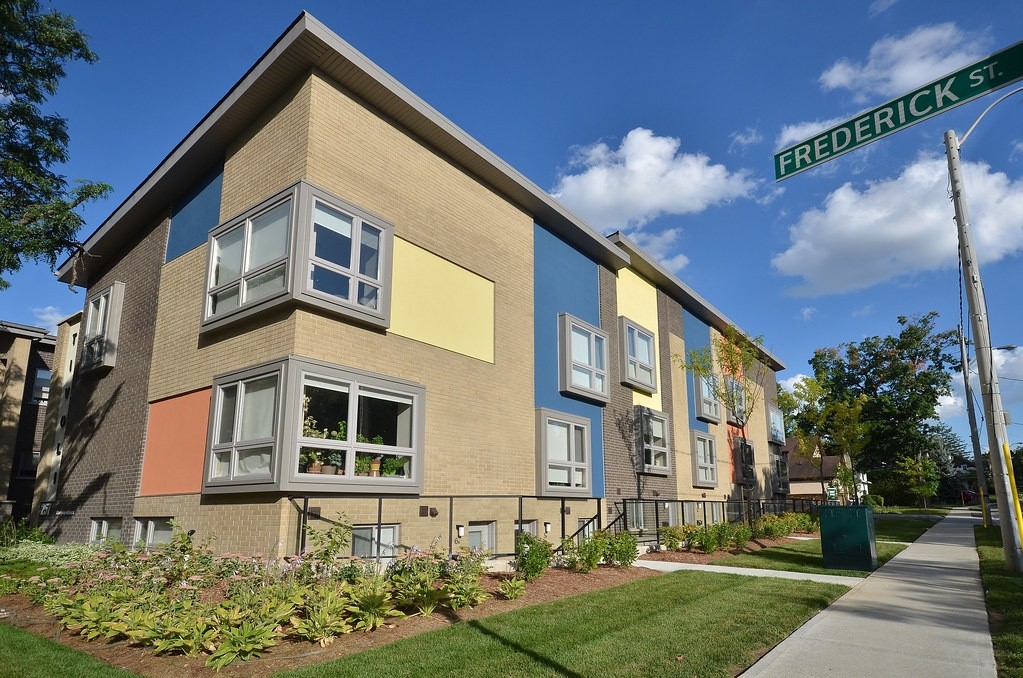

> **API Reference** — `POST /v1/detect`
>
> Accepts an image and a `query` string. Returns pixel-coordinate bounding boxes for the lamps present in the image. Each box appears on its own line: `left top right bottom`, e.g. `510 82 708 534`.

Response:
544 522 552 533
456 525 465 536
697 503 702 508
664 502 668 509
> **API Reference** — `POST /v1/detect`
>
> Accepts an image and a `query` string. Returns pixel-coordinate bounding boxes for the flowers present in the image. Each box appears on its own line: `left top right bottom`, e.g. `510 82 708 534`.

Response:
306 420 327 466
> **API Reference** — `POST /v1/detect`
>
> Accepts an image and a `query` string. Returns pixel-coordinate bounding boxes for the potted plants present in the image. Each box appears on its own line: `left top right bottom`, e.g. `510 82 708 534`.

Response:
334 420 345 474
321 440 342 474
369 435 384 470
356 434 370 476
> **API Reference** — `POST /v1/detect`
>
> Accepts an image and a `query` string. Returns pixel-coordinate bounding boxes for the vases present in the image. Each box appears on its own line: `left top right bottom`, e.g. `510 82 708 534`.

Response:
308 460 321 472
369 469 380 477
